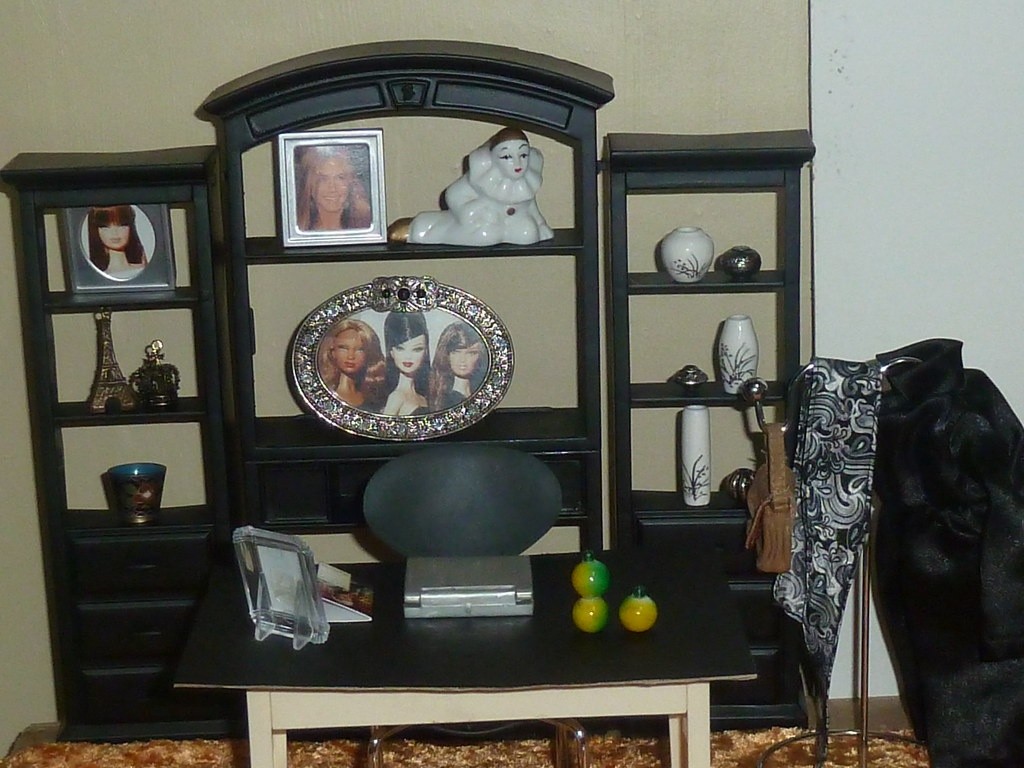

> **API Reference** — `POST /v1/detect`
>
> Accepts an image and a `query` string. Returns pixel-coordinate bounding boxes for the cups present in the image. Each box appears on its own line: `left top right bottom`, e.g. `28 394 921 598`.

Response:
107 461 167 526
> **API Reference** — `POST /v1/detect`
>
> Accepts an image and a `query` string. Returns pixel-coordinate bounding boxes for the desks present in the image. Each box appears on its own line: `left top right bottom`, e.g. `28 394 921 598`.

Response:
175 538 758 768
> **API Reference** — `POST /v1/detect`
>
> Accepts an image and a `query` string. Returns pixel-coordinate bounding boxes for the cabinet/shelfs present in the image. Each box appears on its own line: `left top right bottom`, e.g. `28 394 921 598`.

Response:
0 143 249 741
195 42 615 558
604 128 815 730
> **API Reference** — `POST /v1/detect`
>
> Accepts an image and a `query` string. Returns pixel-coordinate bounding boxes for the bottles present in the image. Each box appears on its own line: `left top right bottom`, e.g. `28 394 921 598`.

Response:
722 245 761 280
619 584 658 632
719 314 758 394
661 227 714 283
573 551 610 633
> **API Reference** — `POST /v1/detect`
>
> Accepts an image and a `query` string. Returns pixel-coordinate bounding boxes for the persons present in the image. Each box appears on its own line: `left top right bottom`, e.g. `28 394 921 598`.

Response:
298 143 371 230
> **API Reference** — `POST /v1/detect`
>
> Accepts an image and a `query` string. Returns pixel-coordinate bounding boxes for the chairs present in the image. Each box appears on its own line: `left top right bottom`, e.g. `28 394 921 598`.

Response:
363 444 590 768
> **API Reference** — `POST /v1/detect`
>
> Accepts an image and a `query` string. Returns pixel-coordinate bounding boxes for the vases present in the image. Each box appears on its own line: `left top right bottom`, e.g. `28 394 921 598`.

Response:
680 405 710 507
718 313 759 395
107 463 167 525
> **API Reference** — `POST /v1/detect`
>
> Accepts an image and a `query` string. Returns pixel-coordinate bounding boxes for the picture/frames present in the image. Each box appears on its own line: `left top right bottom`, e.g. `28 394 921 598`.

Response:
288 276 516 441
55 204 177 295
277 128 388 247
660 226 715 283
232 526 330 651
